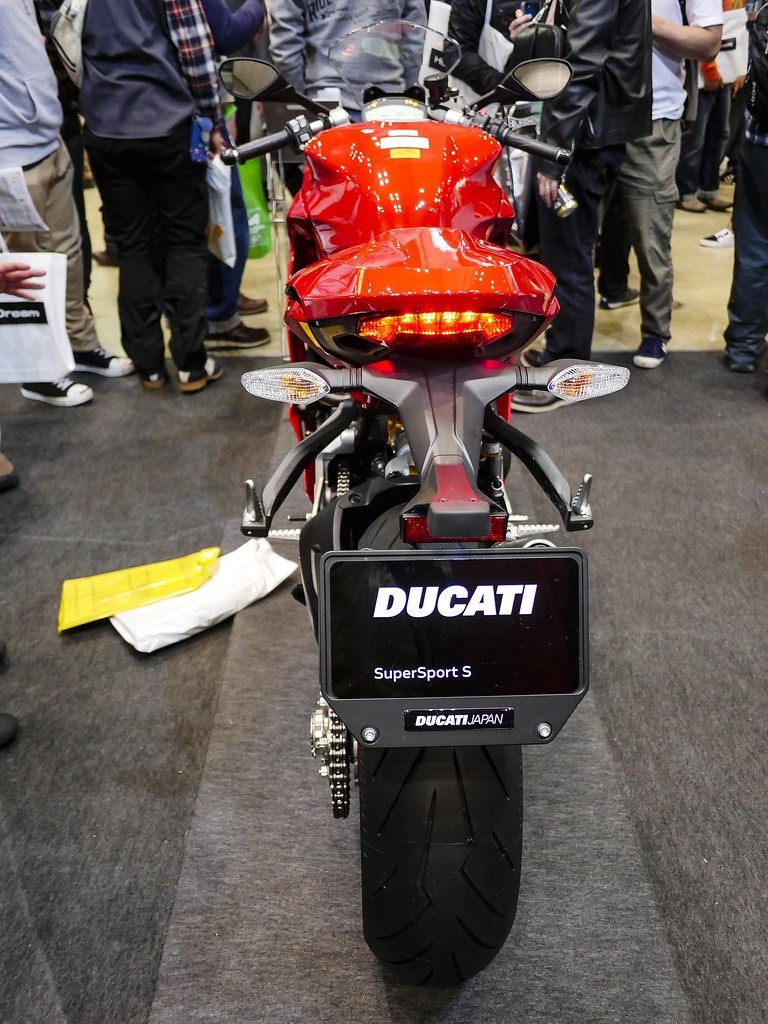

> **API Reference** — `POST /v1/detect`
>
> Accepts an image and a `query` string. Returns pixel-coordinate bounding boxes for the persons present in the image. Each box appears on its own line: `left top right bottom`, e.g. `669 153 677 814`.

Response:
0 2 768 491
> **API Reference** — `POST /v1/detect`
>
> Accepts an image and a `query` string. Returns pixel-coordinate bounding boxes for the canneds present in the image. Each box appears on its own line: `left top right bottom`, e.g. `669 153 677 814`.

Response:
537 176 579 218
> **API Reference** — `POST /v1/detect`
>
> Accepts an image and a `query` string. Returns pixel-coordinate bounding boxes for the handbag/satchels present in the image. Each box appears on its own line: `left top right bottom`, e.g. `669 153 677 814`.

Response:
0 253 75 386
697 8 750 89
201 151 242 268
232 158 273 260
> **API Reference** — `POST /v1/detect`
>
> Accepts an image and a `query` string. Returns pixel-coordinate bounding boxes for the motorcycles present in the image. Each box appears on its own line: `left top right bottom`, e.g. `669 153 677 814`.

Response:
207 20 633 988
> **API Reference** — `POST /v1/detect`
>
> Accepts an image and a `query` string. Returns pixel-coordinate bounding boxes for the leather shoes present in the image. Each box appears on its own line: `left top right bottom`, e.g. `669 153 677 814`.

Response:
509 389 566 415
696 192 733 210
235 293 268 315
202 320 271 351
678 197 706 213
93 250 122 267
520 348 544 369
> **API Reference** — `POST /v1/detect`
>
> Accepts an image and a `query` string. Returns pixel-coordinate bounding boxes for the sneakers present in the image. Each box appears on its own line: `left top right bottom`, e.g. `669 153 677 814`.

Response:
721 335 768 373
630 335 669 370
173 353 225 395
136 365 170 392
696 227 734 248
68 347 135 379
600 288 640 310
18 376 94 411
